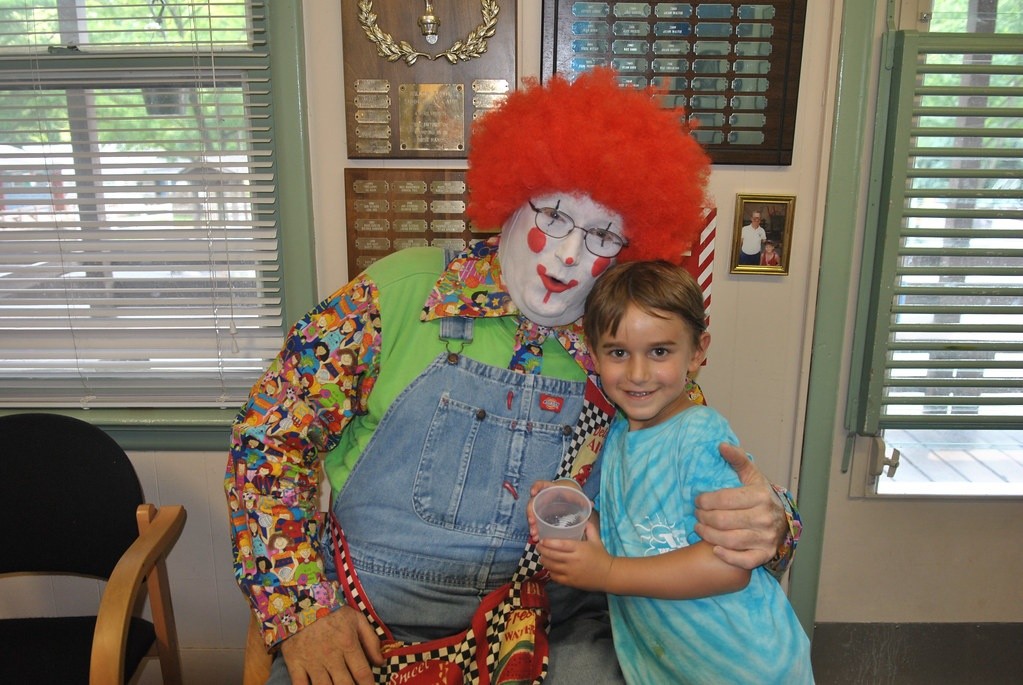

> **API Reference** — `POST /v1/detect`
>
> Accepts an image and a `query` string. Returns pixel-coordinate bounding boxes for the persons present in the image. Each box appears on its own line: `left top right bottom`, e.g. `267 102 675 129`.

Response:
739 210 766 265
224 66 805 685
526 262 816 684
760 240 780 266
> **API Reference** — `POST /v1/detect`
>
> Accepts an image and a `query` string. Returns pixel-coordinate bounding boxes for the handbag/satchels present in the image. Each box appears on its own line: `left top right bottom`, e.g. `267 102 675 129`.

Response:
370 580 550 685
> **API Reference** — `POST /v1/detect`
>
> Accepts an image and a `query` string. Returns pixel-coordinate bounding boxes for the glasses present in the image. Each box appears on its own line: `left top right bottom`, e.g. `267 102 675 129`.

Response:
526 198 630 258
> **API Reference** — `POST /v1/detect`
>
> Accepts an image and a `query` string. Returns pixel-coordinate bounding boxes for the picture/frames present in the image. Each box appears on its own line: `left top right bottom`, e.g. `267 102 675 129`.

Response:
731 193 799 276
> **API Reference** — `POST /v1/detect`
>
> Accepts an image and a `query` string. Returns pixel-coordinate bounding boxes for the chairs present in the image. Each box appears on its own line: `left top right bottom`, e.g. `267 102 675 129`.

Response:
0 413 187 685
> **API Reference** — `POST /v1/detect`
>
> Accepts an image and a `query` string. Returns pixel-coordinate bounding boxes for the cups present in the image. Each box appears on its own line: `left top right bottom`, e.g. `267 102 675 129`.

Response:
532 486 591 551
